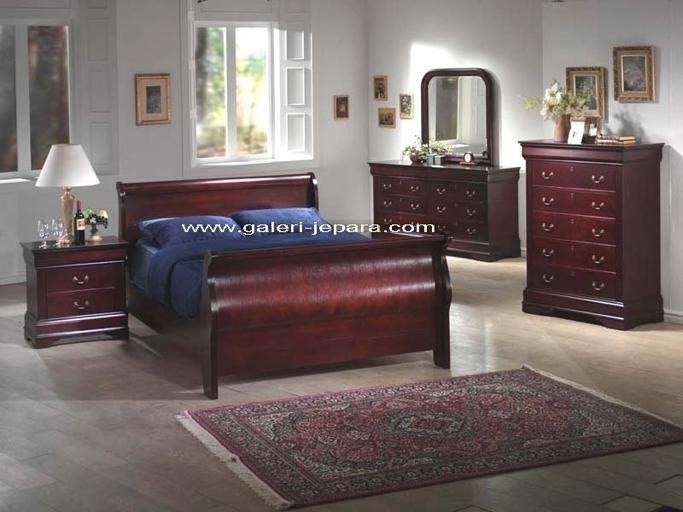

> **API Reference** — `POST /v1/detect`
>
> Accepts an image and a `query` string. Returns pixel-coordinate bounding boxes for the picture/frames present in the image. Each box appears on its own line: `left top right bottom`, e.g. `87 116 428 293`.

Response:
372 75 389 101
612 45 657 103
565 66 606 121
134 72 172 126
398 93 416 119
333 94 350 119
377 106 396 129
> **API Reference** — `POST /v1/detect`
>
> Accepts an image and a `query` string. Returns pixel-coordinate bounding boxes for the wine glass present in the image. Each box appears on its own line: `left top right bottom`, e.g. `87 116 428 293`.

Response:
52 219 64 248
38 219 49 247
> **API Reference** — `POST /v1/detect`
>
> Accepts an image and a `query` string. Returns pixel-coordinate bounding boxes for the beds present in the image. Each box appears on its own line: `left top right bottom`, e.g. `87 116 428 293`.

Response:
113 172 454 400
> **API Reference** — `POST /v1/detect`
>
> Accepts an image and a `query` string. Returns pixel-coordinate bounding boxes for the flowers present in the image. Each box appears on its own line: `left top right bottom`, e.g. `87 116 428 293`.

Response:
521 79 592 122
400 134 452 162
80 207 110 233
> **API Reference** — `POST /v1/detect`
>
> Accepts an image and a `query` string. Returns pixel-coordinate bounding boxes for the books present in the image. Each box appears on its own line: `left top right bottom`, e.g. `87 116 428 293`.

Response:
595 133 636 145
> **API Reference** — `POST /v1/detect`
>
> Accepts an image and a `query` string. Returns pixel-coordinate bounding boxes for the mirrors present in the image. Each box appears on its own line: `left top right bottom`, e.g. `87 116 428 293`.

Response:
420 67 494 166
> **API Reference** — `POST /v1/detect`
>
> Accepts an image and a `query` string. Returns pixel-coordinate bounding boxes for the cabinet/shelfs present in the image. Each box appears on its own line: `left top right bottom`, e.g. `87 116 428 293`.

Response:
517 138 665 331
367 160 520 262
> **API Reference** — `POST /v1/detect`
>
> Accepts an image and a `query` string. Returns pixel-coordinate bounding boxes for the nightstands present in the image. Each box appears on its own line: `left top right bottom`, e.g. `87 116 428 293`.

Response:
19 240 131 349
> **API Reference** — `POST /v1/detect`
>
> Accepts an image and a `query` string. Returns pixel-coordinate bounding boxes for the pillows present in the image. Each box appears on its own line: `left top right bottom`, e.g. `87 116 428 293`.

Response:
137 214 247 262
229 207 336 236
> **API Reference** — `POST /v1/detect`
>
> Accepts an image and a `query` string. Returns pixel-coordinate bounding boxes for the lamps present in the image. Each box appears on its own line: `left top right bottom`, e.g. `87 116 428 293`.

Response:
35 144 100 243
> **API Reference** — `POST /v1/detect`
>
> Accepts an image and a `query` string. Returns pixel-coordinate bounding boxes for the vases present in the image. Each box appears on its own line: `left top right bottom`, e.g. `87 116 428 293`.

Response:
409 154 426 163
553 116 571 142
85 234 102 242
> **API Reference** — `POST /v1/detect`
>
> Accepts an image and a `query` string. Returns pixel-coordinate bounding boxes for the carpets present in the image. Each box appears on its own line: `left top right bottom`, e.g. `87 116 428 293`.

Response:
175 364 682 511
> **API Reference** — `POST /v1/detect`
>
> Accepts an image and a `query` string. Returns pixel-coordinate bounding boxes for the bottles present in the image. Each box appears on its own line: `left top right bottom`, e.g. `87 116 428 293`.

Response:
73 201 85 245
